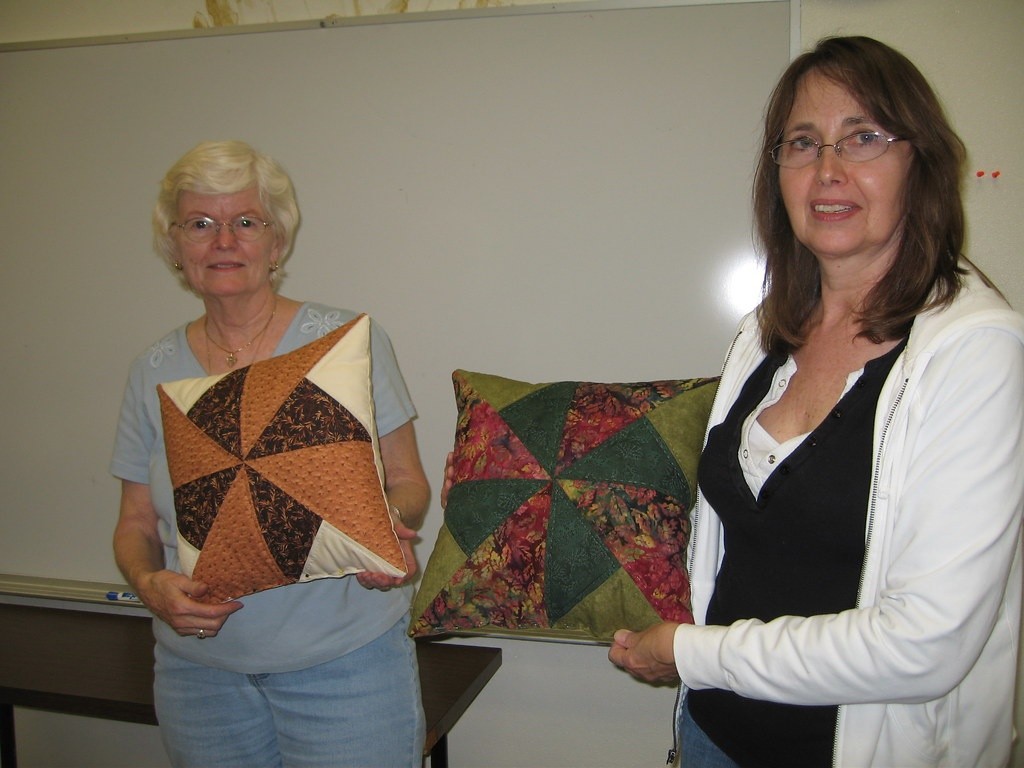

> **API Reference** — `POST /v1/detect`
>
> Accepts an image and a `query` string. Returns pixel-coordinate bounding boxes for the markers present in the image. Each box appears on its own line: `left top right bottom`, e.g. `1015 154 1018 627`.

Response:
106 591 140 602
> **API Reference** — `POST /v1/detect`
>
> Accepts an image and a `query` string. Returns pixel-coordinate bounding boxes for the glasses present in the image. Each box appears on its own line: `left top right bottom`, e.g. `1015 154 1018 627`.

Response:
769 131 909 169
172 216 274 242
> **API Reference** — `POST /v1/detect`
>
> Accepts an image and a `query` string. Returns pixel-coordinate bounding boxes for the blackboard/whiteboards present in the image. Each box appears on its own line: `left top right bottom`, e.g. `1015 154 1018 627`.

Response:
0 0 1023 649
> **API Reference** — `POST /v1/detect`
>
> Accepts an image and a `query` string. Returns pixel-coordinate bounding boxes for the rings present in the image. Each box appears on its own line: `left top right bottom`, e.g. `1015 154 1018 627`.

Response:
197 628 206 639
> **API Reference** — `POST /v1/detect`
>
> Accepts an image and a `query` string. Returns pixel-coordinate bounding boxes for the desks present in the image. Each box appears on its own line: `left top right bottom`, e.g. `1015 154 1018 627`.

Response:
0 602 503 768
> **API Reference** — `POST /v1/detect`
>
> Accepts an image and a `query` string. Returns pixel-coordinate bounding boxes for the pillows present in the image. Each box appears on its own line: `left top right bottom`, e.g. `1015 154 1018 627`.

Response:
407 370 722 643
157 314 408 604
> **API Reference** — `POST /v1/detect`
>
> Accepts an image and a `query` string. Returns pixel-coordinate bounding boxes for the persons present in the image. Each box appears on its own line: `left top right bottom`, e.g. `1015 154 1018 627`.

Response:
608 36 1024 768
110 139 430 768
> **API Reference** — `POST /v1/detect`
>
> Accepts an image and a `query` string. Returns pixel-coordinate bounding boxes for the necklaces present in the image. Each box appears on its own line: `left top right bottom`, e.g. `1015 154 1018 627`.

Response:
204 296 277 368
